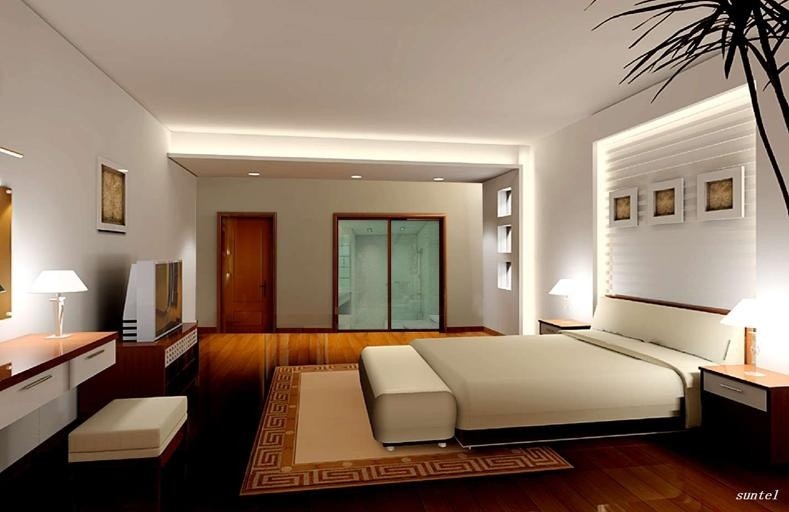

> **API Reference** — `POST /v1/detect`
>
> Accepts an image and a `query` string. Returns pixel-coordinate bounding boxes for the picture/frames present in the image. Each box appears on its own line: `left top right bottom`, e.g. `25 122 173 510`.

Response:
608 187 638 228
696 166 745 222
95 156 128 233
648 178 684 226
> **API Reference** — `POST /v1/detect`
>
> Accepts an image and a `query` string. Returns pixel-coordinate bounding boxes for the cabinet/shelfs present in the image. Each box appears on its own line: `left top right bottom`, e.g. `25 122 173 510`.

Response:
77 322 200 423
0 331 118 476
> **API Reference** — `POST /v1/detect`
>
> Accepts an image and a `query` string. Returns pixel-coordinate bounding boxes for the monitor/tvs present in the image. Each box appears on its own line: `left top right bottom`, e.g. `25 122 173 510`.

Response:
120 257 184 342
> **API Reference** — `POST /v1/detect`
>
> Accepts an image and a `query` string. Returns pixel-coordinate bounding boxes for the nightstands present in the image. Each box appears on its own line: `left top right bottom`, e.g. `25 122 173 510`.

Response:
538 320 591 335
698 364 789 466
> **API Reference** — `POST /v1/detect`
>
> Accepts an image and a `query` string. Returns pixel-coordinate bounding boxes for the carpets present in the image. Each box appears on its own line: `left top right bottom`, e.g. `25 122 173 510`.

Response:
238 362 574 496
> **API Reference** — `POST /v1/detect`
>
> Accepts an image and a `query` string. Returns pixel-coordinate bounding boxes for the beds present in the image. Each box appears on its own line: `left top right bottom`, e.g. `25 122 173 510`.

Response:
358 294 745 451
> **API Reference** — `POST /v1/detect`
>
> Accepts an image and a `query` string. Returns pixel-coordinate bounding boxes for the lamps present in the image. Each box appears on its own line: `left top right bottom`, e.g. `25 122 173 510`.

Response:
720 295 782 378
30 270 88 339
548 279 586 322
